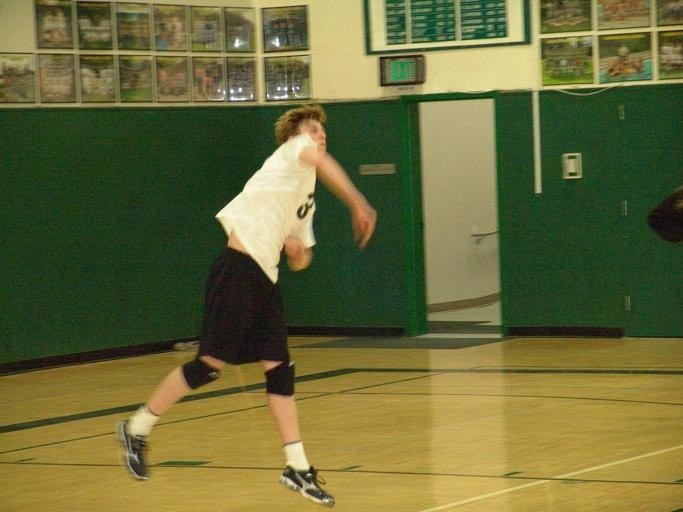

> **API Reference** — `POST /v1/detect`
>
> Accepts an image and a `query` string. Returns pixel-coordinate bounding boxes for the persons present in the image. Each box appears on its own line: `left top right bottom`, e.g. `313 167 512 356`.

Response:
115 106 377 506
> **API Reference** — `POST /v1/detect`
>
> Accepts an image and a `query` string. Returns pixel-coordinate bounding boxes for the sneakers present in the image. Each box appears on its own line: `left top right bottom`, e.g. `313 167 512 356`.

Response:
281 465 335 506
118 419 147 480
